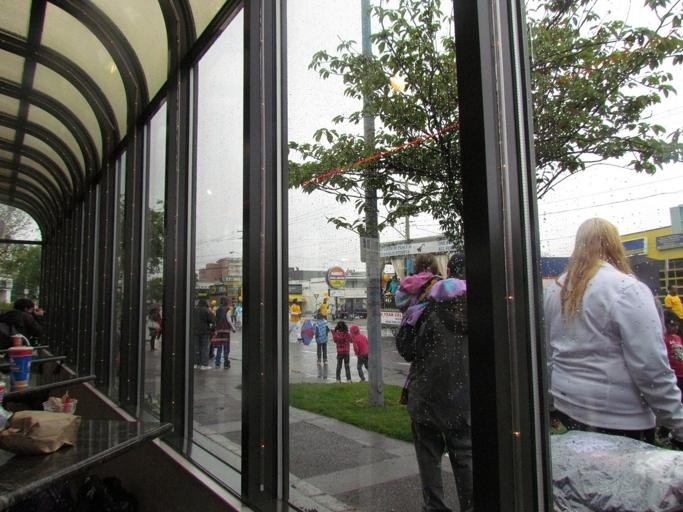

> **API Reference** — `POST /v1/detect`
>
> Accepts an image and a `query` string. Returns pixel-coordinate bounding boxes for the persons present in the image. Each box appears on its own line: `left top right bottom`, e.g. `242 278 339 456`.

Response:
396 253 473 510
663 310 682 392
0 298 45 348
144 294 370 381
544 220 682 445
664 286 682 320
395 253 444 406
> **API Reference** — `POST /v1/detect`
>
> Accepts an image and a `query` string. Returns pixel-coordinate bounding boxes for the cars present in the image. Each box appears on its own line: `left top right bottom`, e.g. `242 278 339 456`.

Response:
10 335 23 346
7 346 34 389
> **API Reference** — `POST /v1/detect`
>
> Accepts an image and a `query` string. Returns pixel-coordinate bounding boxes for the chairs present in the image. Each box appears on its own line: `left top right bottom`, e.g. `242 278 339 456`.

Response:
224 362 230 369
200 366 212 370
215 364 219 369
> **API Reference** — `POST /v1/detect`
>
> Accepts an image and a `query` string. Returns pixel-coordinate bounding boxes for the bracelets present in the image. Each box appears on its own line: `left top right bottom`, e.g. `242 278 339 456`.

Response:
43 399 76 416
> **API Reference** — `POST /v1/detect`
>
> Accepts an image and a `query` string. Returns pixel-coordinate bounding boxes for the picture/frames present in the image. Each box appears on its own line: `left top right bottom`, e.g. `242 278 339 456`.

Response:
0 410 175 511
0 355 67 373
0 370 97 411
0 343 49 354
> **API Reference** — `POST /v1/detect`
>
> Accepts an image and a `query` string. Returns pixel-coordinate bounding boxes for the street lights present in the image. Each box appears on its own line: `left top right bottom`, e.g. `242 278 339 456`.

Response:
0 410 81 455
